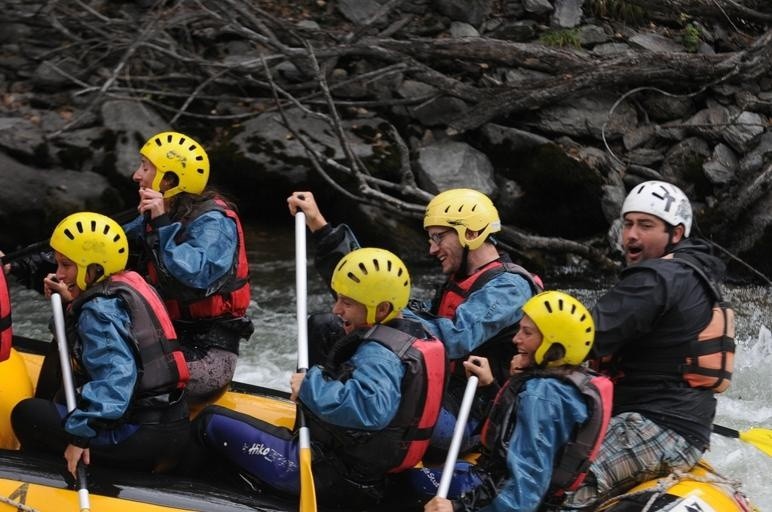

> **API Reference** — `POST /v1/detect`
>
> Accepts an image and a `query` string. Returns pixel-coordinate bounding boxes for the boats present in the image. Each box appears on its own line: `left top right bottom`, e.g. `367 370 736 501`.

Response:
0 337 757 512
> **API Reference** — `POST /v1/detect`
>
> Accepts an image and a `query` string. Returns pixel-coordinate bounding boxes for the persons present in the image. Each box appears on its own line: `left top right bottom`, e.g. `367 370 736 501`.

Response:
9 210 196 481
25 129 251 398
189 191 447 497
375 290 615 512
557 178 735 512
306 187 544 454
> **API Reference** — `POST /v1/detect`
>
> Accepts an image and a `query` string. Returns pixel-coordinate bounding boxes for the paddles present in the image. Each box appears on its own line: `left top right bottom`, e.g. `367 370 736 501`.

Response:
711 423 772 460
294 195 318 512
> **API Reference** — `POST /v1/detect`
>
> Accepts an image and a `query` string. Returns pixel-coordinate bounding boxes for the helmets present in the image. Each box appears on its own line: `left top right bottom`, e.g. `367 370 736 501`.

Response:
421 187 503 254
328 245 412 331
518 287 596 371
48 210 130 293
138 129 212 200
617 177 694 240
